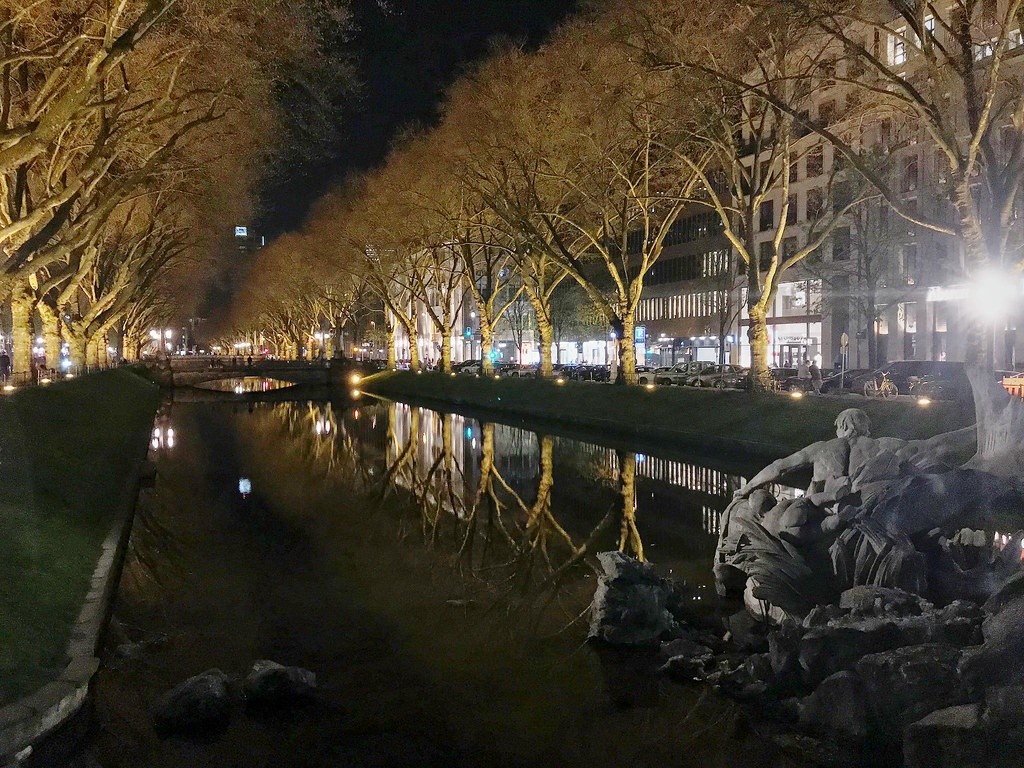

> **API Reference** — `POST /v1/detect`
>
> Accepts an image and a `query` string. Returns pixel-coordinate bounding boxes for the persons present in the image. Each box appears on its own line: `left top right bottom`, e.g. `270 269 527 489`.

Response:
798 360 812 378
809 360 823 396
247 355 252 365
119 357 127 364
784 360 789 367
217 357 221 368
732 407 977 499
0 350 10 382
211 356 214 367
232 356 236 364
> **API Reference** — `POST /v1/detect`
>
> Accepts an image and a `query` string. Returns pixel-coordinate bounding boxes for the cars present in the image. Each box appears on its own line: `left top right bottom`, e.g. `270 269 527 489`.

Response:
772 369 876 394
348 358 411 371
451 360 538 379
852 360 1024 395
552 364 610 383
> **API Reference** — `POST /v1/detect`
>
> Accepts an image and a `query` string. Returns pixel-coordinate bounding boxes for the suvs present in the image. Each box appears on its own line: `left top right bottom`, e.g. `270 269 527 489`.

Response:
634 361 750 389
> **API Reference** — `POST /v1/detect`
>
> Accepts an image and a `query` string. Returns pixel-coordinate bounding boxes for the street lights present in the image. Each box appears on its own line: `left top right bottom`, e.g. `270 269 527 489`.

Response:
467 327 473 360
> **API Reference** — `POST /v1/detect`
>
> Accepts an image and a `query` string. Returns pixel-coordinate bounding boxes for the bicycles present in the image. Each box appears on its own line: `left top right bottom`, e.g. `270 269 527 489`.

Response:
863 370 898 401
